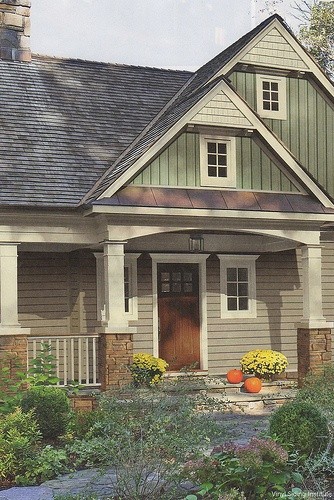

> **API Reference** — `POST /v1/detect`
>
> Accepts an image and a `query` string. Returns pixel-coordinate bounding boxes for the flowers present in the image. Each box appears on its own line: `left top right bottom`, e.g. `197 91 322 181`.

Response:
129 352 166 387
242 348 288 382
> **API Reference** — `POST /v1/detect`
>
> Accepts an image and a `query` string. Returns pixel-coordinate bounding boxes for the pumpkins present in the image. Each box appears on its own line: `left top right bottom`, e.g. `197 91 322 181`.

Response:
244 376 261 393
227 369 243 384
